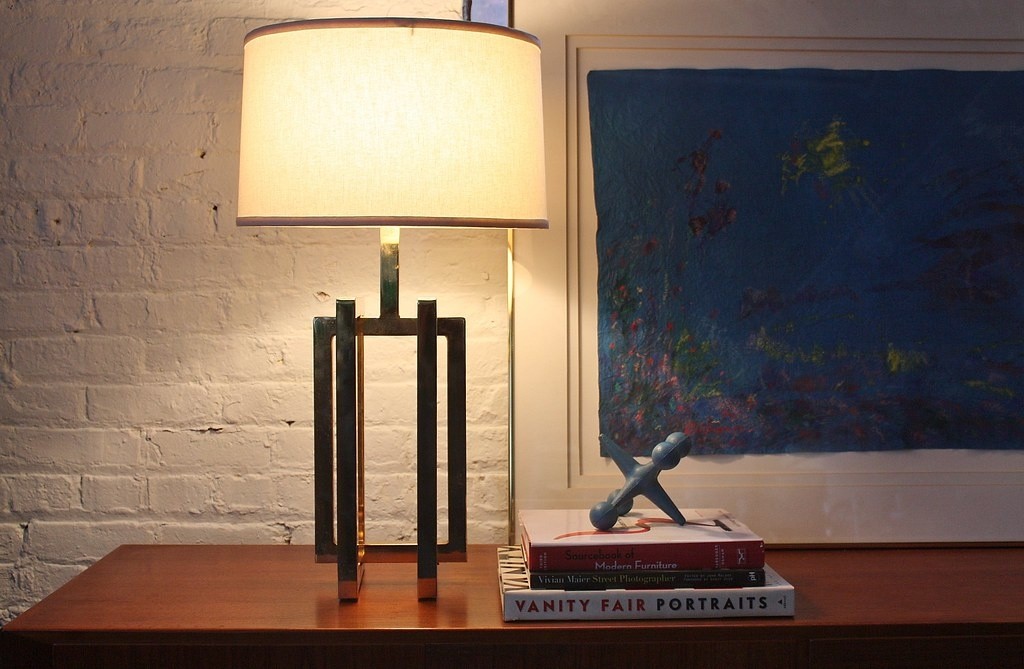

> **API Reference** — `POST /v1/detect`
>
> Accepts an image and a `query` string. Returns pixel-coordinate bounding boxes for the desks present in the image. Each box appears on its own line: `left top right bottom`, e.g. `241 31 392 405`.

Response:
1 544 1023 669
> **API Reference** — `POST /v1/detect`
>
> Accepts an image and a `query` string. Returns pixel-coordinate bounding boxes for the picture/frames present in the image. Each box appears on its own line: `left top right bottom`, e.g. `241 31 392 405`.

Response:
512 1 1023 546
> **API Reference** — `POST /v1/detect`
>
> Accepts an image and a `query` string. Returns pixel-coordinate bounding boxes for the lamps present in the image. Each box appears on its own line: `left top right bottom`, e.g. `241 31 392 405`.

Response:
236 17 549 602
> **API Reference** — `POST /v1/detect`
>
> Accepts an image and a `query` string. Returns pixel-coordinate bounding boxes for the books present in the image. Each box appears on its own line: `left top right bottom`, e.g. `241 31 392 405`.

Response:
496 507 796 622
